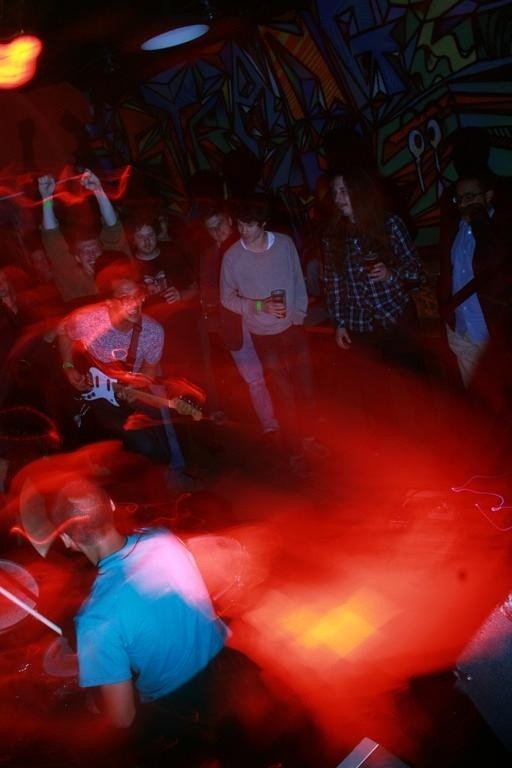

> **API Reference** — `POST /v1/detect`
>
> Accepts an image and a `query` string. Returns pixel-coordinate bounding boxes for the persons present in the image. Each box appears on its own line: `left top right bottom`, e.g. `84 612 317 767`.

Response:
197 210 282 435
371 170 507 440
218 204 337 463
323 173 431 376
3 169 197 489
47 478 262 730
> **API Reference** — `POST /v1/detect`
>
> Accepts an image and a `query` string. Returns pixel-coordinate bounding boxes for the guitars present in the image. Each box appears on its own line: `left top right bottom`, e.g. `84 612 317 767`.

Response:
81 366 203 421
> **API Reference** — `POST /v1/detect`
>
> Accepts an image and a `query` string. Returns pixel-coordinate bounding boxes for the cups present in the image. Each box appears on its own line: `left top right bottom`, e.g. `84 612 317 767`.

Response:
364 254 378 280
270 288 287 319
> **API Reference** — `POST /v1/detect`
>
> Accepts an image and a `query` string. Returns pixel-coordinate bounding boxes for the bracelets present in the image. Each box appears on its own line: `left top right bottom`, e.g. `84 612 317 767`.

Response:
43 201 53 208
255 300 261 313
60 360 74 369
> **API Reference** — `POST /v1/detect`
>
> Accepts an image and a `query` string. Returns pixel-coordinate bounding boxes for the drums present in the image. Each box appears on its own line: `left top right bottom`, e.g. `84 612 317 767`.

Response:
1 560 41 647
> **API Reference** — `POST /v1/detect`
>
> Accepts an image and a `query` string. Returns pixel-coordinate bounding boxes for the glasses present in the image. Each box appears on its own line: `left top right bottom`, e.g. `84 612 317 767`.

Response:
110 292 146 304
452 193 484 204
